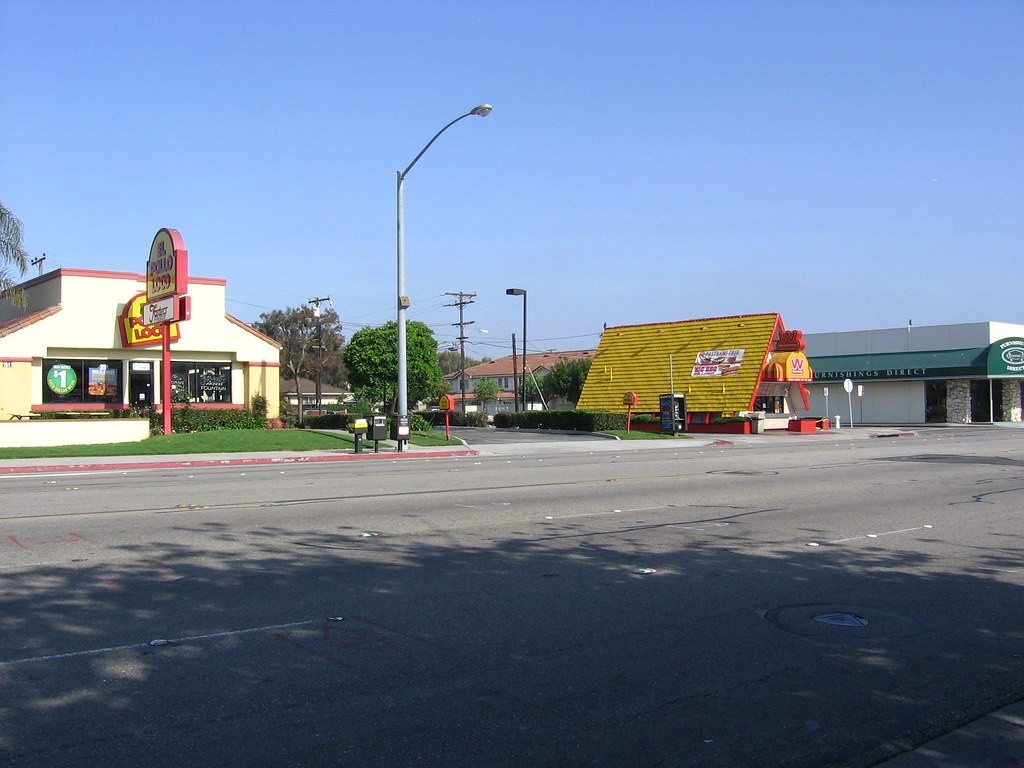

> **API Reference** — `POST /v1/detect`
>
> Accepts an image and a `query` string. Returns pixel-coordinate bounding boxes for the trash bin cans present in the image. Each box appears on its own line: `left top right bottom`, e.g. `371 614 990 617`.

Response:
390 416 411 453
751 412 764 435
366 416 387 454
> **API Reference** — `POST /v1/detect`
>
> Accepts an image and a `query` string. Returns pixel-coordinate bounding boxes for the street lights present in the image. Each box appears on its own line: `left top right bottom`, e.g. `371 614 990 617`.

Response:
505 288 527 410
395 104 493 415
